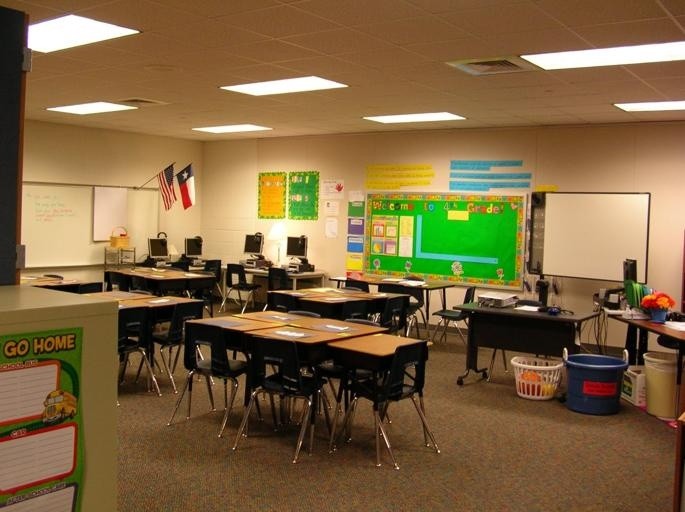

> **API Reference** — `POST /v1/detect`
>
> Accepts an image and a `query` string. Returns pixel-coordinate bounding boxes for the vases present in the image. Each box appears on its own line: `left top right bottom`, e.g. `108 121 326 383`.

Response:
648 309 668 323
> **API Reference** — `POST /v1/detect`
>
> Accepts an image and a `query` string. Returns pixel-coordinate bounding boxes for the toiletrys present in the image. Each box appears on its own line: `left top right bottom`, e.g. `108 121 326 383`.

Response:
536 273 549 305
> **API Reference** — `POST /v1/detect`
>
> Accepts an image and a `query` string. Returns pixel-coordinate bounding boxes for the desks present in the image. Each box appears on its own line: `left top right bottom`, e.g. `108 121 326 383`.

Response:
448 299 603 391
35 258 221 394
222 266 325 312
592 294 685 421
329 275 454 316
265 287 409 332
190 309 429 382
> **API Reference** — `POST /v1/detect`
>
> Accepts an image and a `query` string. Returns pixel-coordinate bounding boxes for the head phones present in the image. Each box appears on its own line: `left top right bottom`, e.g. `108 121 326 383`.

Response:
297 234 308 249
195 236 202 246
157 232 167 246
253 232 264 246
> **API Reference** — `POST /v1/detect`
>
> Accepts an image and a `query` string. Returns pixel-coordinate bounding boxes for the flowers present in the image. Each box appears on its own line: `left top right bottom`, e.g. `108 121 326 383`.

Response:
641 291 675 311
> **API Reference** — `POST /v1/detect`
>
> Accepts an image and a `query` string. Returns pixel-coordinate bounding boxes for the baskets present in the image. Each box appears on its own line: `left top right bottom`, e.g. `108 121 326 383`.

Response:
110 225 130 248
509 356 564 400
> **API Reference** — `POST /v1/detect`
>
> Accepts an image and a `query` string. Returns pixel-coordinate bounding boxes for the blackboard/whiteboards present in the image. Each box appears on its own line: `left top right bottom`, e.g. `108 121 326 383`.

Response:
16 181 162 269
527 191 651 284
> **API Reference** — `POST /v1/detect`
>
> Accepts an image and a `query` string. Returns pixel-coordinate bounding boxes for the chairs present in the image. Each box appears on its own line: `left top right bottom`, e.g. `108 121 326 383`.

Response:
264 267 290 313
431 286 478 343
217 263 261 315
337 345 442 469
164 322 263 439
347 278 419 343
278 310 382 425
380 296 411 338
398 277 427 333
232 336 336 461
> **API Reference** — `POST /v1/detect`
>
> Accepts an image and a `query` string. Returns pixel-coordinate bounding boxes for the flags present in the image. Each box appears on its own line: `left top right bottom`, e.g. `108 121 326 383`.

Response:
177 164 195 211
156 163 177 212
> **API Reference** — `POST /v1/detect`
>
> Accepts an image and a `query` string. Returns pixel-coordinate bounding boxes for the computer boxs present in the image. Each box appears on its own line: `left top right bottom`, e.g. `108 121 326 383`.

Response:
147 261 171 267
181 260 208 266
281 264 314 272
239 260 272 268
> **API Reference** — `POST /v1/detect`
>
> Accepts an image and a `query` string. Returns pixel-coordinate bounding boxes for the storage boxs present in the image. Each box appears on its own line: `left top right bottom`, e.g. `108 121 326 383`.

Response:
621 364 648 407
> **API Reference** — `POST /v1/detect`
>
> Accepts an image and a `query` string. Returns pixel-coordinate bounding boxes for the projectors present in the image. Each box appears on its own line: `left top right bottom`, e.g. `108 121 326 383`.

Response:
478 292 519 308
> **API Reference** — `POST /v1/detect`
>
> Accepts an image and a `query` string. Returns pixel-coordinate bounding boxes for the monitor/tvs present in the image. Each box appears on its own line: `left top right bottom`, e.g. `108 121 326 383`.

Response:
286 236 307 264
623 257 637 290
243 234 264 260
148 238 169 261
185 237 202 260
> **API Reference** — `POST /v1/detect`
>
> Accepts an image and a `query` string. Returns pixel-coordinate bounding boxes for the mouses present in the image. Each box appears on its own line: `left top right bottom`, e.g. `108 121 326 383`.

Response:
292 270 298 273
259 266 266 269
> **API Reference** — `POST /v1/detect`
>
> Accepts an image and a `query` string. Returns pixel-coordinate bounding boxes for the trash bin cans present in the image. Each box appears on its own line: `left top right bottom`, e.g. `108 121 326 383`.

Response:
643 352 685 418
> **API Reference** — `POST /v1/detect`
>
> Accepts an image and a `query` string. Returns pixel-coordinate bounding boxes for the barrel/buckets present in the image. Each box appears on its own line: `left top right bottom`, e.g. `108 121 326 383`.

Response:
562 354 629 415
643 352 679 417
